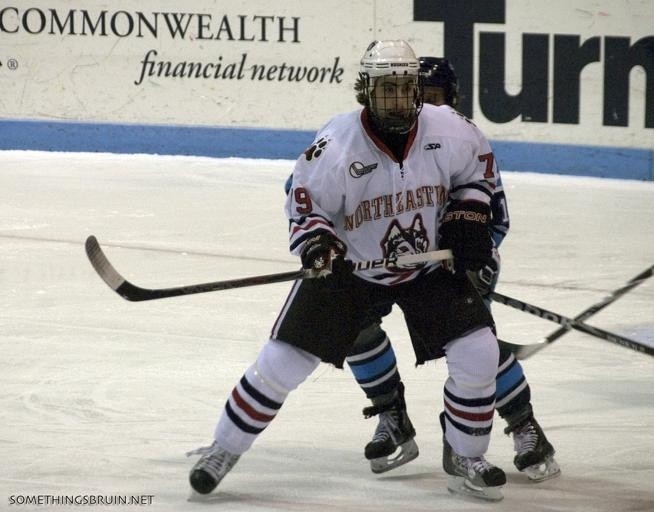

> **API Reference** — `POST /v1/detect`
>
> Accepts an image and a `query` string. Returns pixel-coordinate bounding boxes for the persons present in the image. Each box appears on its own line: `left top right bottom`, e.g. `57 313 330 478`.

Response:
184 37 507 496
344 55 555 473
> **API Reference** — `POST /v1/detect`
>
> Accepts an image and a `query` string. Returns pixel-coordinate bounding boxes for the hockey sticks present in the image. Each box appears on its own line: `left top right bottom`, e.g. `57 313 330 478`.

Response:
498 265 654 361
87 236 454 302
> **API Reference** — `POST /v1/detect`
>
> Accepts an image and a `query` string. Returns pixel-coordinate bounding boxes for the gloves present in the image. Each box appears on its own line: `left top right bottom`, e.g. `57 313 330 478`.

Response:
449 222 494 272
301 231 347 270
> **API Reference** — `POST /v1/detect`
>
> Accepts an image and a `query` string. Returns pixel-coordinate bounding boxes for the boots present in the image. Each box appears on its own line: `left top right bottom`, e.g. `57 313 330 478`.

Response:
439 411 507 490
505 412 556 472
363 382 416 460
190 441 241 495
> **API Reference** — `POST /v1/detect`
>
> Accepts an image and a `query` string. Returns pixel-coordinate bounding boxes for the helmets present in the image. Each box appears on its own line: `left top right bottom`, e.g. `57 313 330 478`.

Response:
361 38 425 134
416 55 460 107
